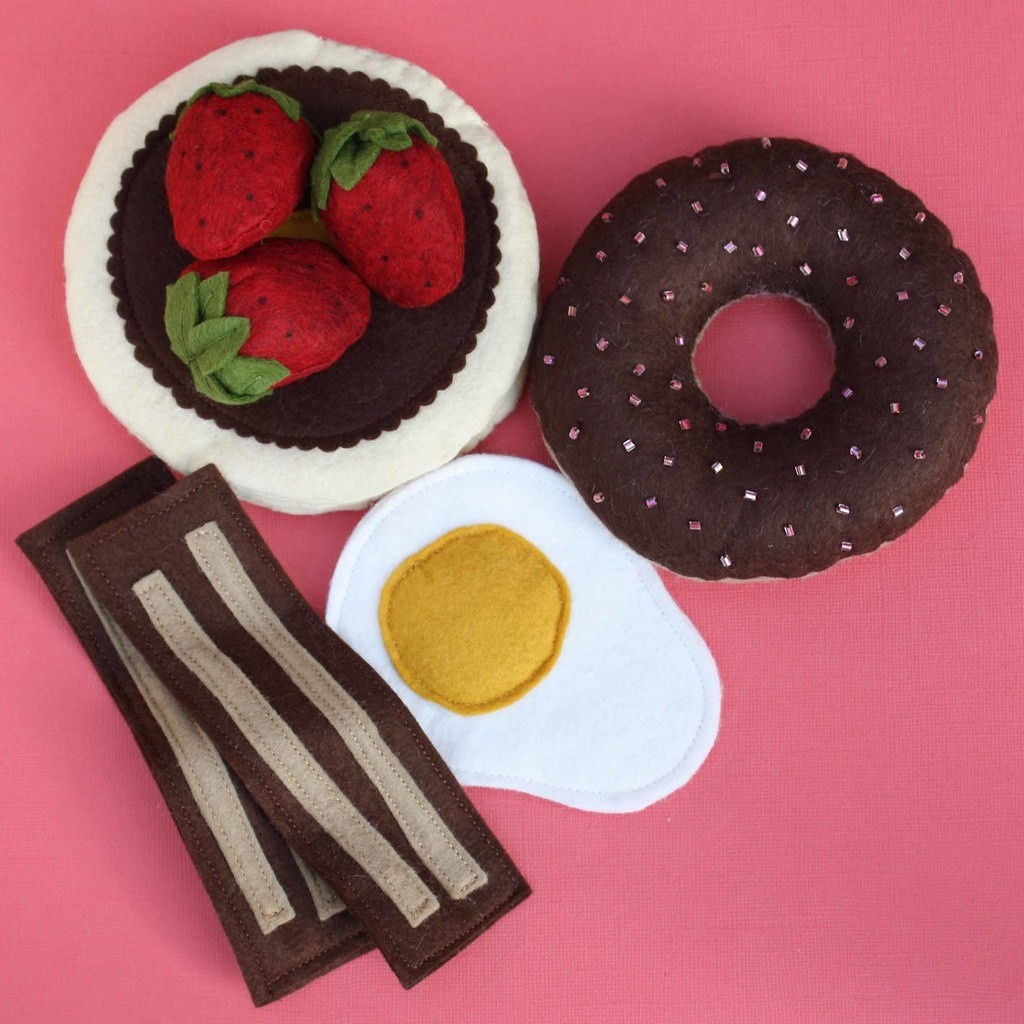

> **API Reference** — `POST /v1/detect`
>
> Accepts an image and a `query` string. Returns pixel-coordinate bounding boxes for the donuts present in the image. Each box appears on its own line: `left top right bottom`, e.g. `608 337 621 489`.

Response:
527 137 1001 581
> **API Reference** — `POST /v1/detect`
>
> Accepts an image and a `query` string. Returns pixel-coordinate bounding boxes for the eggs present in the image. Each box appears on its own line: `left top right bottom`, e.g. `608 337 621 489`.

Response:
320 453 723 816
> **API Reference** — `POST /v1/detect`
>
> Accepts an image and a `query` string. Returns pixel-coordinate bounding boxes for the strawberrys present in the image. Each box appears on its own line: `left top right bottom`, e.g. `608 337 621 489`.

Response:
164 82 466 406
